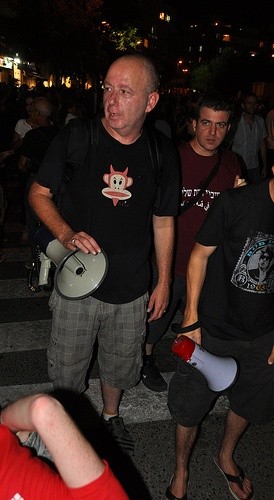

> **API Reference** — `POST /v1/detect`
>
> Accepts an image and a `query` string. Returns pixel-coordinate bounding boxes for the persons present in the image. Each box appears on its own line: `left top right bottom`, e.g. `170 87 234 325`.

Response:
0 85 274 500
27 54 186 461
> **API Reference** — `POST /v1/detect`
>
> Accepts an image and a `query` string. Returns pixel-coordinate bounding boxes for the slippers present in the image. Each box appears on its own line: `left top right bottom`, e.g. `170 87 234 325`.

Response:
213 452 252 500
166 474 191 500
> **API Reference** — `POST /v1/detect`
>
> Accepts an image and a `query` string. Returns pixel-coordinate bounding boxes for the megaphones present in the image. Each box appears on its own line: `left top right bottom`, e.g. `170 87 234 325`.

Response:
171 334 239 394
33 226 108 301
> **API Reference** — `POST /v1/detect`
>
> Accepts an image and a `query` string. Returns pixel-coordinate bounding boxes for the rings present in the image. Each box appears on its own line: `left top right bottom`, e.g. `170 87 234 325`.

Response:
73 239 76 245
164 310 166 312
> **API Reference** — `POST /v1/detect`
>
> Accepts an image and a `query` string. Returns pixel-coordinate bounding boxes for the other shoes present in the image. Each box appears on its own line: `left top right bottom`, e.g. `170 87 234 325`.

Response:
101 411 134 457
142 354 168 391
